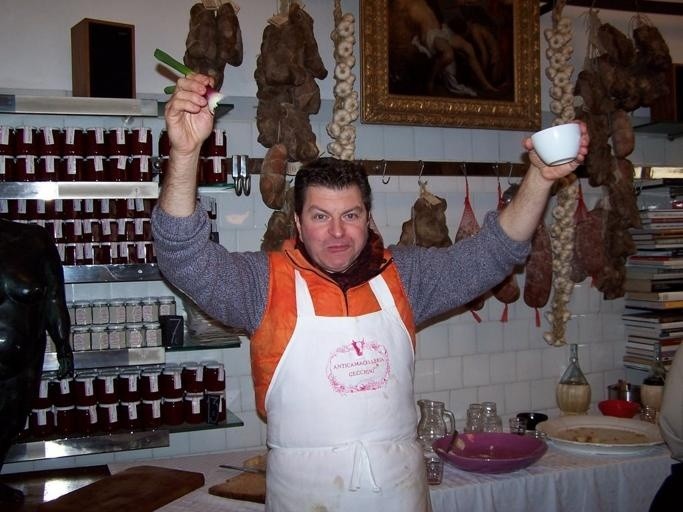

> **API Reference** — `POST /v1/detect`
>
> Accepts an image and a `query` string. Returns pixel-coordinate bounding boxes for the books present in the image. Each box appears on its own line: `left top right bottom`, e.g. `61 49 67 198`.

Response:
620 202 683 375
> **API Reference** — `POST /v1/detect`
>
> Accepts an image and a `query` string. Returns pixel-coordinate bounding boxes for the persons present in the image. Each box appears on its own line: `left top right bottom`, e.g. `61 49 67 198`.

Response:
0 218 74 503
388 1 501 98
150 74 589 511
647 342 683 511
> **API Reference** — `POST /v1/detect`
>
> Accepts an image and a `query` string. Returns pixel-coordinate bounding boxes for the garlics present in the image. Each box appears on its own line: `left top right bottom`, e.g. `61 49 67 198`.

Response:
335 82 352 97
551 240 562 254
563 32 573 46
545 67 557 80
350 111 359 121
546 312 554 323
552 206 565 218
343 13 354 21
342 149 354 161
339 129 356 145
338 21 354 36
550 223 561 237
551 100 563 114
559 177 569 190
549 34 564 50
565 107 573 111
562 227 575 239
564 83 575 94
564 200 578 216
546 48 554 58
554 72 569 87
561 94 574 107
560 293 569 303
338 41 353 58
347 75 355 84
344 97 359 112
564 280 574 294
571 174 577 180
344 144 355 150
560 216 574 228
552 260 562 272
560 233 572 244
562 111 565 120
560 250 573 262
351 91 357 99
565 110 575 122
326 123 340 139
550 53 566 70
342 125 355 131
562 262 571 267
558 17 573 34
333 110 351 126
563 46 574 60
550 86 562 100
346 55 355 67
334 62 350 81
552 302 558 309
327 143 342 156
560 266 573 278
562 311 570 322
543 332 556 345
544 28 555 40
552 118 564 126
553 277 566 292
567 185 580 199
331 32 338 40
345 36 355 43
565 64 574 78
564 242 573 251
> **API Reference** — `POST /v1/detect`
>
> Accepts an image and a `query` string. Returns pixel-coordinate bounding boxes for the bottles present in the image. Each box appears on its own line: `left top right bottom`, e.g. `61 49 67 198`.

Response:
205 128 227 155
163 398 183 424
160 370 185 398
97 375 119 404
74 379 96 405
40 155 61 181
45 330 57 352
134 198 145 217
87 128 109 156
126 197 135 217
74 300 92 326
143 323 163 347
92 243 110 265
0 155 17 182
117 401 140 435
110 242 119 261
98 198 116 218
126 323 144 348
75 243 84 264
16 411 31 439
140 398 163 431
117 218 135 241
0 125 16 155
125 298 143 322
44 219 64 241
17 126 44 154
11 218 44 232
556 344 591 415
57 244 75 265
96 403 119 433
55 406 77 439
640 341 666 423
15 155 40 182
84 217 103 241
31 199 50 221
183 395 206 424
159 156 170 187
52 199 69 219
76 404 98 436
130 154 153 182
142 297 159 323
107 326 125 349
64 127 87 154
140 373 162 399
109 127 134 156
117 198 128 218
134 218 154 240
0 199 11 219
132 128 153 155
205 392 226 424
109 156 135 182
33 378 52 408
207 156 228 187
86 157 111 182
68 326 91 351
92 298 108 325
119 242 136 262
197 156 206 187
65 301 75 326
62 157 87 181
53 378 74 407
40 127 66 156
70 199 83 217
184 366 206 393
90 326 109 349
84 243 93 263
109 298 125 324
84 198 97 218
159 129 171 155
144 199 153 217
205 364 226 391
62 219 84 243
12 198 30 219
136 241 154 263
100 218 118 241
30 408 55 437
158 296 177 316
118 374 141 402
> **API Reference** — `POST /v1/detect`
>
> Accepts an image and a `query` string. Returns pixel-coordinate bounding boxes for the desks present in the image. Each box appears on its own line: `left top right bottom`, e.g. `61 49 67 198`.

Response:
17 444 681 511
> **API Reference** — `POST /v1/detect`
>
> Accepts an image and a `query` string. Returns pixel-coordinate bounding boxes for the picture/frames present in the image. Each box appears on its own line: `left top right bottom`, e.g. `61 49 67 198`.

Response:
360 0 541 133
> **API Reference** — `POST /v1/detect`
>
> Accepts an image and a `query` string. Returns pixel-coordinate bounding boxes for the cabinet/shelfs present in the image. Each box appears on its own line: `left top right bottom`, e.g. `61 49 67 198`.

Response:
0 95 243 466
623 165 683 370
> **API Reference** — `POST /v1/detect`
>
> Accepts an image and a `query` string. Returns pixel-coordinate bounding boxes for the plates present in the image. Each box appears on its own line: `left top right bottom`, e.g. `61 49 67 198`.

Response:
536 412 665 457
431 430 550 477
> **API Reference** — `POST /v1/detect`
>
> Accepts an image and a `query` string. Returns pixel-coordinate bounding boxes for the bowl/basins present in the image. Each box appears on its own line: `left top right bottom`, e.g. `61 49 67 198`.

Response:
530 122 583 168
